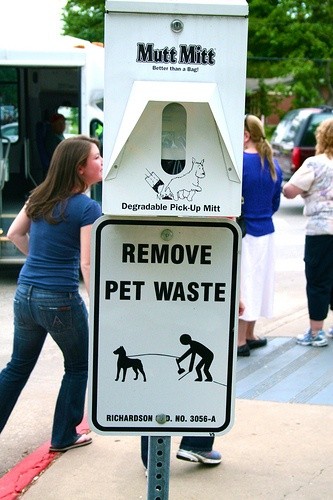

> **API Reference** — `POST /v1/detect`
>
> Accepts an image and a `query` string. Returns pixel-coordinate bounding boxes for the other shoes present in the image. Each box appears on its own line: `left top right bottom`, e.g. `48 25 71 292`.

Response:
237 336 266 356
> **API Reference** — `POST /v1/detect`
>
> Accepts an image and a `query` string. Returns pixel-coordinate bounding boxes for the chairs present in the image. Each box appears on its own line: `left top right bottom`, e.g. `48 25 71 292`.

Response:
30 122 52 179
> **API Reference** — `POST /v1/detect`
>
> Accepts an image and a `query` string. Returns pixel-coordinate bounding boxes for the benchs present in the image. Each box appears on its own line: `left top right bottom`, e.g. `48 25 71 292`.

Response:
0 133 11 212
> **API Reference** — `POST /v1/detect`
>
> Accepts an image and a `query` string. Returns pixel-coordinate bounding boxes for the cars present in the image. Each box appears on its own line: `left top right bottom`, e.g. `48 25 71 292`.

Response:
1 122 19 143
270 107 333 182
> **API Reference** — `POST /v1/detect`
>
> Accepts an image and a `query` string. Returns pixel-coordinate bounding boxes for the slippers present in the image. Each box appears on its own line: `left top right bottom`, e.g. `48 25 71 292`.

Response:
49 433 92 451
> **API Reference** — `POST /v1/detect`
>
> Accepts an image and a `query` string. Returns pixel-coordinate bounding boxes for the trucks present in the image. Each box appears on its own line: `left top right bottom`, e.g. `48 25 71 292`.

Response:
0 44 103 264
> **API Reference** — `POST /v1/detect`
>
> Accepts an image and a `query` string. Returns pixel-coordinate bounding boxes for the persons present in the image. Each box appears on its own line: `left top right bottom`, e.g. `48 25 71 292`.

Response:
283 119 333 348
0 135 103 451
48 115 68 149
140 216 245 477
237 114 282 357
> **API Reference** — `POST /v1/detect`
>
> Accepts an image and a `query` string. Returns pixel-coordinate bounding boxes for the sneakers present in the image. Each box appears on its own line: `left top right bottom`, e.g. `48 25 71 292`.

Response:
176 444 222 464
296 328 328 347
326 326 333 337
